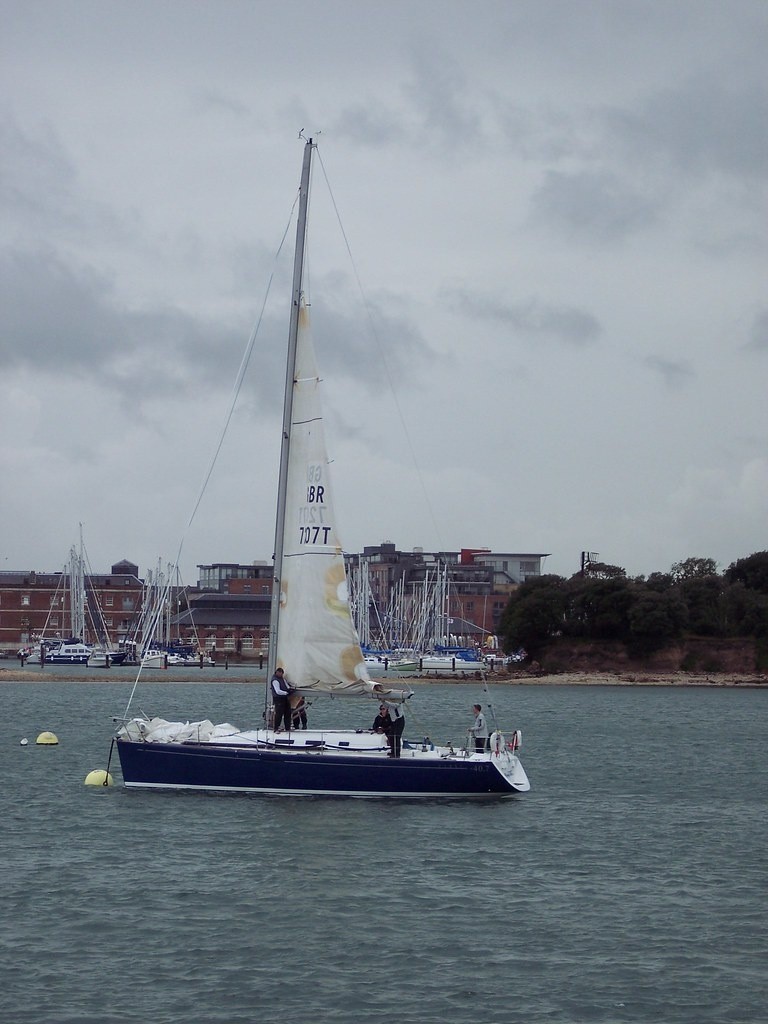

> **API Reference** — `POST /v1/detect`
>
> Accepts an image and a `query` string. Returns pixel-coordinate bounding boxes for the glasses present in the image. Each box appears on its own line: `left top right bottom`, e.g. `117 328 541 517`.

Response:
379 708 386 710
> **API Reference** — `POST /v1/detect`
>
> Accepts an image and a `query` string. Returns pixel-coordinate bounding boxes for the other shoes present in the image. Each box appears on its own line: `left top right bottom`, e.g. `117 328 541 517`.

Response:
274 729 280 733
283 729 295 732
387 753 400 758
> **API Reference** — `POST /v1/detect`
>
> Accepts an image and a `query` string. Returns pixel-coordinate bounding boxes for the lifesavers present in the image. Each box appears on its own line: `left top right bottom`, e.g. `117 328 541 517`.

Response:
490 732 506 754
513 730 523 750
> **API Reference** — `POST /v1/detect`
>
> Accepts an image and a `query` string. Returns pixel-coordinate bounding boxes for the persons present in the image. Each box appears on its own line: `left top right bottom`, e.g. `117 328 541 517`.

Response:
373 699 405 758
468 704 487 753
271 668 292 734
293 696 307 729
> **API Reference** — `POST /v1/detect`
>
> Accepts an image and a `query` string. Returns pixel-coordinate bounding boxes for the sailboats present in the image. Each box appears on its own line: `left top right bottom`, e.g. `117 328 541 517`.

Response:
344 553 523 677
113 128 531 796
27 522 215 668
